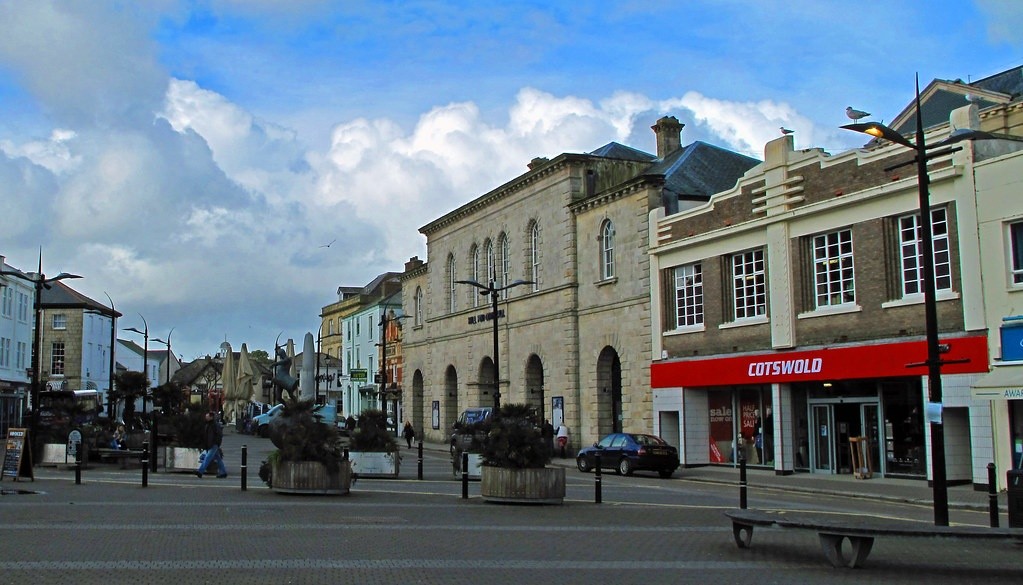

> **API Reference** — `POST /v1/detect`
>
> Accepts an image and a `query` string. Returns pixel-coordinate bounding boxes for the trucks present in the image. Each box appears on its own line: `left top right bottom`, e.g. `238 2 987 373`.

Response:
252 404 337 438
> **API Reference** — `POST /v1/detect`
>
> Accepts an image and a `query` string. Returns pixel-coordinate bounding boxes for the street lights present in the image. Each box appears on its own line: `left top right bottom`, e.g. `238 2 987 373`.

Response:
123 313 148 428
839 72 997 528
314 319 342 401
325 348 332 403
274 331 296 406
454 278 538 413
0 246 84 455
149 326 174 382
378 300 413 448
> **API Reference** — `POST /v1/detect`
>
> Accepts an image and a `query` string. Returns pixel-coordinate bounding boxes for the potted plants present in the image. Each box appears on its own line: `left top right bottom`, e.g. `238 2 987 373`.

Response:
165 402 215 468
105 370 150 451
36 396 91 463
261 406 357 492
341 407 400 475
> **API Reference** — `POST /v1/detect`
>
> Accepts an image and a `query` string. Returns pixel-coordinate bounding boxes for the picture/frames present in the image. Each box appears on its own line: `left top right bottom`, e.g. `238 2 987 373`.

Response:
551 396 564 435
431 401 439 430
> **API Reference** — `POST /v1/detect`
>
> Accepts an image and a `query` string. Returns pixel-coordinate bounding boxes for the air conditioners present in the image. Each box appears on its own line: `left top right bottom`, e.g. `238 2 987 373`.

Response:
454 404 569 498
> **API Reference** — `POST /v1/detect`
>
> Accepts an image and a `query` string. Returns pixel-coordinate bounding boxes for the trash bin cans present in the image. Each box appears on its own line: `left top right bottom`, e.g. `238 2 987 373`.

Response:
1007 470 1023 528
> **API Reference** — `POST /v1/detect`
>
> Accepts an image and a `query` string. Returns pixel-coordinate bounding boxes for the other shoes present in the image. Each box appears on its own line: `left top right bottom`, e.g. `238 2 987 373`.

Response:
193 470 202 479
216 473 227 478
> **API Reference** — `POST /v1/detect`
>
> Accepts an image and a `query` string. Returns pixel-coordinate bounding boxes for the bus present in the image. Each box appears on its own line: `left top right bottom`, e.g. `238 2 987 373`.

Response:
38 389 99 426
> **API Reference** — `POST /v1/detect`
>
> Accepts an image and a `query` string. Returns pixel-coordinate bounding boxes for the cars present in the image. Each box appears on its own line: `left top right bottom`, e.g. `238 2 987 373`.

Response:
576 432 680 478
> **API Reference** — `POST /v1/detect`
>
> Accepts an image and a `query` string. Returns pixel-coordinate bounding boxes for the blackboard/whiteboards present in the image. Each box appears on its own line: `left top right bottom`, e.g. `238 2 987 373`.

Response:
1 428 33 477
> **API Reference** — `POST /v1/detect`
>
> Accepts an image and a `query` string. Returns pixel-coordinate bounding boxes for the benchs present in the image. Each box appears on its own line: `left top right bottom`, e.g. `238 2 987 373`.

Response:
725 507 1022 565
91 447 152 469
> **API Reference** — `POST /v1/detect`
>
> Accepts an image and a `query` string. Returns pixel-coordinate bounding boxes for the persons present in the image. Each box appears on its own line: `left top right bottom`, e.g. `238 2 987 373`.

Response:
193 412 228 479
731 432 744 458
404 421 415 449
269 346 299 407
752 409 767 465
544 419 554 455
554 423 568 459
111 433 121 450
765 407 774 462
753 427 765 464
112 425 127 450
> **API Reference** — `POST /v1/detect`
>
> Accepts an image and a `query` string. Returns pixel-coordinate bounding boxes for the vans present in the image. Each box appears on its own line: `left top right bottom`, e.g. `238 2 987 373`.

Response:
451 407 494 458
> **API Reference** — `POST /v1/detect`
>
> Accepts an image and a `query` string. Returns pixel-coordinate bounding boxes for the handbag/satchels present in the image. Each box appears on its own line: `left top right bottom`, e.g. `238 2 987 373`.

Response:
198 450 208 463
413 437 415 443
